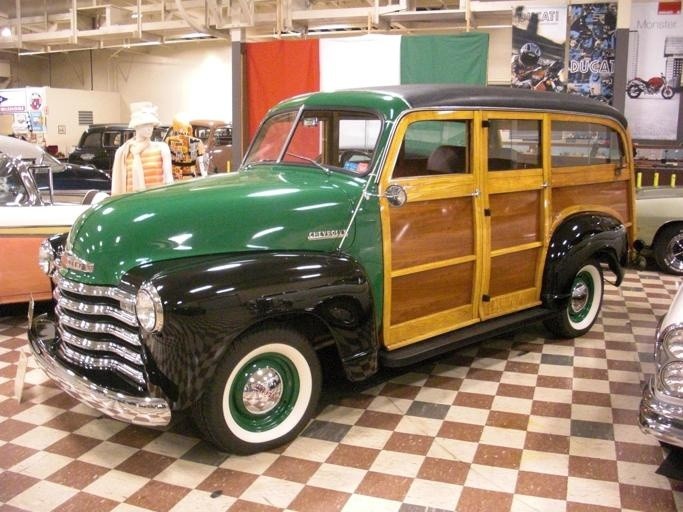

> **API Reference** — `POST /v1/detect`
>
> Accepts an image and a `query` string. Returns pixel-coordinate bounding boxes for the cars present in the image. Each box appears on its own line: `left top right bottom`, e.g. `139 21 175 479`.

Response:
636 280 682 450
633 186 682 275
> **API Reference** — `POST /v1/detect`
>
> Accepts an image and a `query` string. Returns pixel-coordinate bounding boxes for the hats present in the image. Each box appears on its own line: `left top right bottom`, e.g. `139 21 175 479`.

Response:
128 102 162 129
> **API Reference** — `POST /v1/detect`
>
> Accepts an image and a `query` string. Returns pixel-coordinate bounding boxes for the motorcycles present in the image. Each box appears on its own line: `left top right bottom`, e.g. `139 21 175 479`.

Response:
626 72 674 100
520 62 566 93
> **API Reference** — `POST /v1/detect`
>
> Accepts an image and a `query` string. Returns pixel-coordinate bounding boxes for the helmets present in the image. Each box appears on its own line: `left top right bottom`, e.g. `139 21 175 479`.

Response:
520 42 541 66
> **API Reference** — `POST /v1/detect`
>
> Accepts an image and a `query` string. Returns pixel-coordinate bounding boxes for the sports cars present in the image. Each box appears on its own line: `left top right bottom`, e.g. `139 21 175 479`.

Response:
9 155 111 194
0 153 111 309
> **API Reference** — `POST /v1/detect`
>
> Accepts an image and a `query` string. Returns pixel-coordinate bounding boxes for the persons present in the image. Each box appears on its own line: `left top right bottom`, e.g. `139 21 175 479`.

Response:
110 101 174 194
163 112 207 181
511 43 560 88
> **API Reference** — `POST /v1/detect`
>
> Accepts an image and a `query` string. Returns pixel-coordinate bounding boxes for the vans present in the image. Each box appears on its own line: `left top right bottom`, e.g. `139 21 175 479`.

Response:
24 84 636 455
66 123 172 169
186 120 231 173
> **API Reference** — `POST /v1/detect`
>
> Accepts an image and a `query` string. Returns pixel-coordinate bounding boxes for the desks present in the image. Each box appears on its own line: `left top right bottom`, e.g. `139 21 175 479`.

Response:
0 205 89 305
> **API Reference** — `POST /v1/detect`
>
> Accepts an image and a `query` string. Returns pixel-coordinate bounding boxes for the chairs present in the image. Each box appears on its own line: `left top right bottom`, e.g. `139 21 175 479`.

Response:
426 144 467 173
